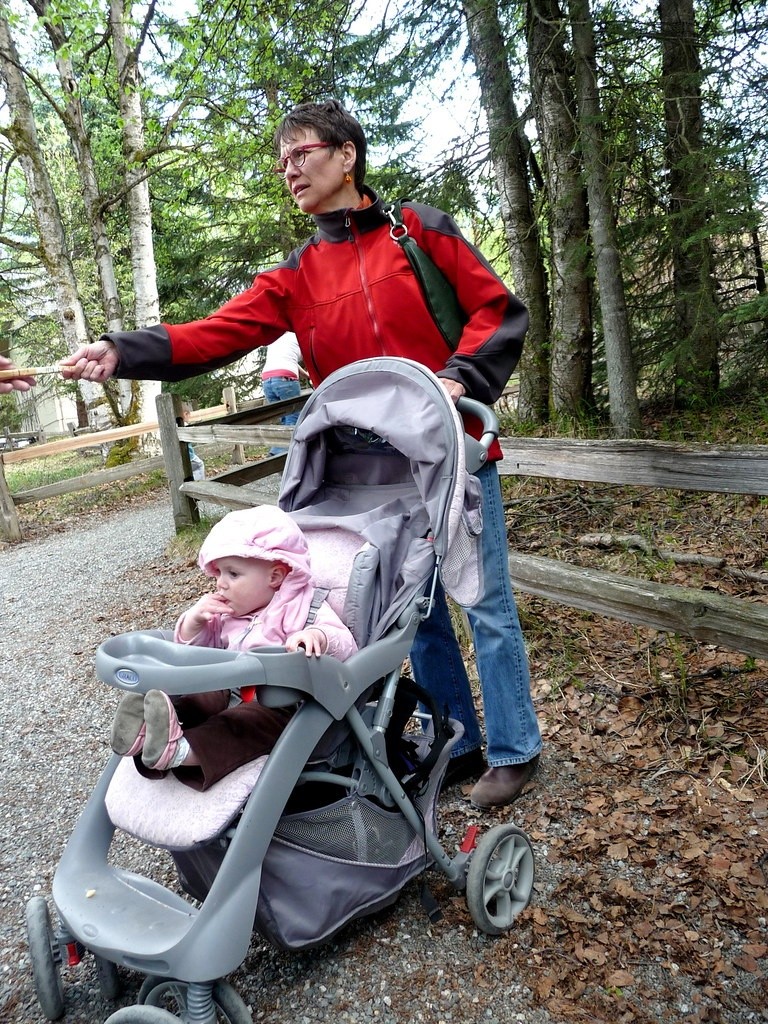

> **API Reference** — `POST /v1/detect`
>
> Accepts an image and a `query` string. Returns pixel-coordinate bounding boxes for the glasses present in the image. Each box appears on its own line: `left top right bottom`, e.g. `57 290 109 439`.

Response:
272 140 337 181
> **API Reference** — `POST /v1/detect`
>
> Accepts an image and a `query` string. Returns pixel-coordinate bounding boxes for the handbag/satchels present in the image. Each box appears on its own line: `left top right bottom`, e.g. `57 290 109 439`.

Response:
383 197 467 352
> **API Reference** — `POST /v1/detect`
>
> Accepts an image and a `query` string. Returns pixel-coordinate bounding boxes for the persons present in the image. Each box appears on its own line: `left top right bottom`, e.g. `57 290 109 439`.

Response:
261 331 302 457
110 504 358 792
182 402 205 483
61 102 543 808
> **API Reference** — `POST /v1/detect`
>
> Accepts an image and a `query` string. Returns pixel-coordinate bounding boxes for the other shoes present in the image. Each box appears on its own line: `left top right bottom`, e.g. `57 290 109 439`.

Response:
140 688 184 771
109 691 148 757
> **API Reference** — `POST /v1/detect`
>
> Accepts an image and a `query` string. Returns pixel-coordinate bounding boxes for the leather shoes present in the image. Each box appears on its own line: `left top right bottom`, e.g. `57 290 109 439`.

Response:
433 747 486 794
469 751 539 812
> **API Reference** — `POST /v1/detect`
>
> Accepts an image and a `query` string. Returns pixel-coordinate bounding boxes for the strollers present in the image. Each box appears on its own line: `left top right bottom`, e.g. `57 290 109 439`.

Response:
24 339 549 1023
